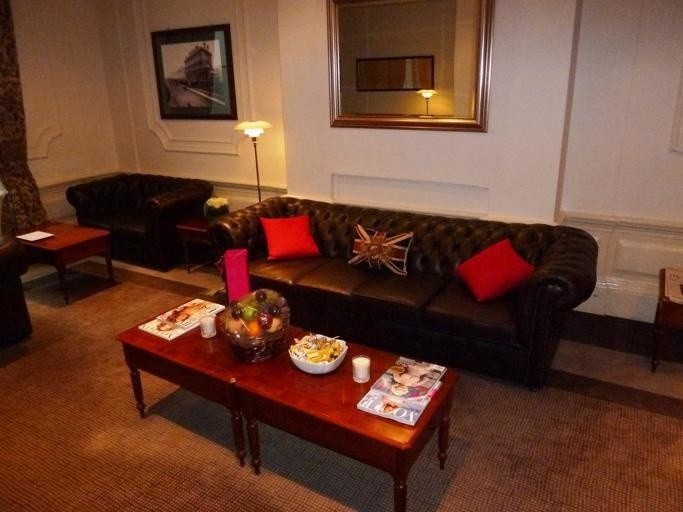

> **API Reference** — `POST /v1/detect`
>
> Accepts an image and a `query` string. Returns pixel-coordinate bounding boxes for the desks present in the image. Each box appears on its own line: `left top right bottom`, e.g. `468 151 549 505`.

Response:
173 216 212 275
649 266 683 373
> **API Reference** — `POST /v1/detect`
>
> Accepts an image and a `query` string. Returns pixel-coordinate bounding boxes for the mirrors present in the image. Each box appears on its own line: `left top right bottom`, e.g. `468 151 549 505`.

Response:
327 0 498 133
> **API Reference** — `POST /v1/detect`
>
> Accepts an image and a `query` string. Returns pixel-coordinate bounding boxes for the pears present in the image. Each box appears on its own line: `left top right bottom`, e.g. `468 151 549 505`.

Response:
223 318 245 336
265 317 282 333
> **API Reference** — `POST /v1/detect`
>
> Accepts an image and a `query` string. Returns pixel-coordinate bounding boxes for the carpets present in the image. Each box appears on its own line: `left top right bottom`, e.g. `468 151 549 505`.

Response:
1 257 683 512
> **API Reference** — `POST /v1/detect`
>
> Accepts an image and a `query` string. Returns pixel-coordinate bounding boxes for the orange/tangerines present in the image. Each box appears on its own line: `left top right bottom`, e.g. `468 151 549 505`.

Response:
245 321 263 338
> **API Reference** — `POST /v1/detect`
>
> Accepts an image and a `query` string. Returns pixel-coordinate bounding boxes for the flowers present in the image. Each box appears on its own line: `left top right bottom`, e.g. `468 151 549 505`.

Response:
206 196 228 208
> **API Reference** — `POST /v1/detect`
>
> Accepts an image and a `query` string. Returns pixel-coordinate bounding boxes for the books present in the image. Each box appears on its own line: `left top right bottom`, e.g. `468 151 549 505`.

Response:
355 380 442 426
15 231 54 242
370 356 447 405
138 318 201 342
155 298 225 330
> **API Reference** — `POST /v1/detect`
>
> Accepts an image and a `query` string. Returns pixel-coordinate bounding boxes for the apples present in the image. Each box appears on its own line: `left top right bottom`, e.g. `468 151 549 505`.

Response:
242 305 260 322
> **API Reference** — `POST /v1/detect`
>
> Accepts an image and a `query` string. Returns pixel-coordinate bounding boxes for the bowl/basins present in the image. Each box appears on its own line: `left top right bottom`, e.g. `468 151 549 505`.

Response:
219 324 283 362
289 334 347 374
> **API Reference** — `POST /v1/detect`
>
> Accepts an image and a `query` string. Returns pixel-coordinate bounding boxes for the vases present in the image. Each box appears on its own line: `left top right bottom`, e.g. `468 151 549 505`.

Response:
206 205 229 223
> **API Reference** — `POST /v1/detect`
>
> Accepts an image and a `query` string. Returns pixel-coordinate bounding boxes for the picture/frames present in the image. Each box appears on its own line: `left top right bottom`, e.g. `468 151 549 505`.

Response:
354 54 436 92
150 22 240 121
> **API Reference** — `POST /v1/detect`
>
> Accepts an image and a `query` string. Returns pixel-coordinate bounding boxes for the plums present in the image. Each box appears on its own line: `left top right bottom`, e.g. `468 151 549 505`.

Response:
255 290 267 302
268 305 280 316
231 307 242 319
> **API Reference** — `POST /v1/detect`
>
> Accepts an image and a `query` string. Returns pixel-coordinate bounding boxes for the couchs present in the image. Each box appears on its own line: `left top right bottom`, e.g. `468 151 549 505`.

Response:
0 233 33 353
207 195 601 394
64 171 213 273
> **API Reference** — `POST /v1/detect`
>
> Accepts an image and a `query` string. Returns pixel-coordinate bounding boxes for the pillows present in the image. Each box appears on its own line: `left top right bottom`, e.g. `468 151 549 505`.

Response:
258 213 323 262
346 222 416 279
451 238 539 304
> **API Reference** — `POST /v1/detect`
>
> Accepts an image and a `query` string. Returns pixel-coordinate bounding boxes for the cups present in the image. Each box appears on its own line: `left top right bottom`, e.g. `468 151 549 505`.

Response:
351 354 371 384
198 316 216 338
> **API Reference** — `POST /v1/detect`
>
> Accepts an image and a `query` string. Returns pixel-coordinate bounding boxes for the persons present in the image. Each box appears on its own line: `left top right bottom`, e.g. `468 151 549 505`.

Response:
380 393 399 414
158 321 176 331
389 361 435 396
167 301 206 323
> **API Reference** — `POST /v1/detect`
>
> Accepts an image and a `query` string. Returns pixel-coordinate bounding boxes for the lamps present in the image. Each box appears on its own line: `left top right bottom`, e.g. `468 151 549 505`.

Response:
415 87 437 114
232 119 272 204
